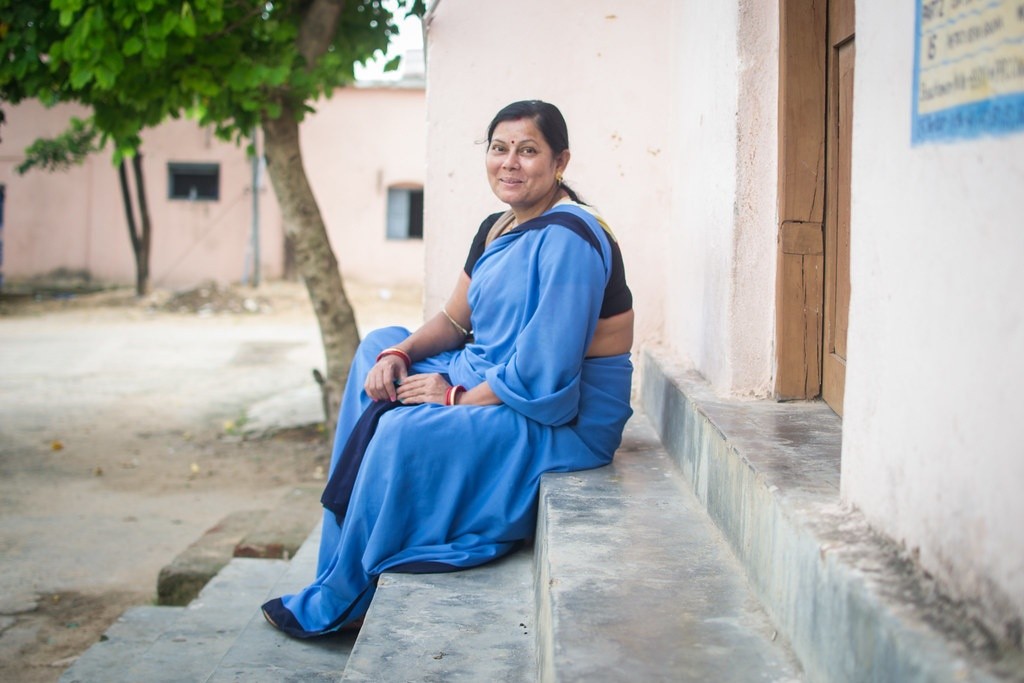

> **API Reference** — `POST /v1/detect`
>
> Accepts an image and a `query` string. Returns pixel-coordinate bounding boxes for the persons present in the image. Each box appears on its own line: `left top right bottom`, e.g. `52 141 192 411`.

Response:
259 100 635 641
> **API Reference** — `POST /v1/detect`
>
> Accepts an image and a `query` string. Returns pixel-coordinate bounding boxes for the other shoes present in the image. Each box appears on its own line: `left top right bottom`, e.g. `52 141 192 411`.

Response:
261 598 364 639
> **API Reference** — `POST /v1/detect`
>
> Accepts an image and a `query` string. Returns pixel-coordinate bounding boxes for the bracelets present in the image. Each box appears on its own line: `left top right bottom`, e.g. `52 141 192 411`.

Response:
446 385 467 405
375 348 412 371
